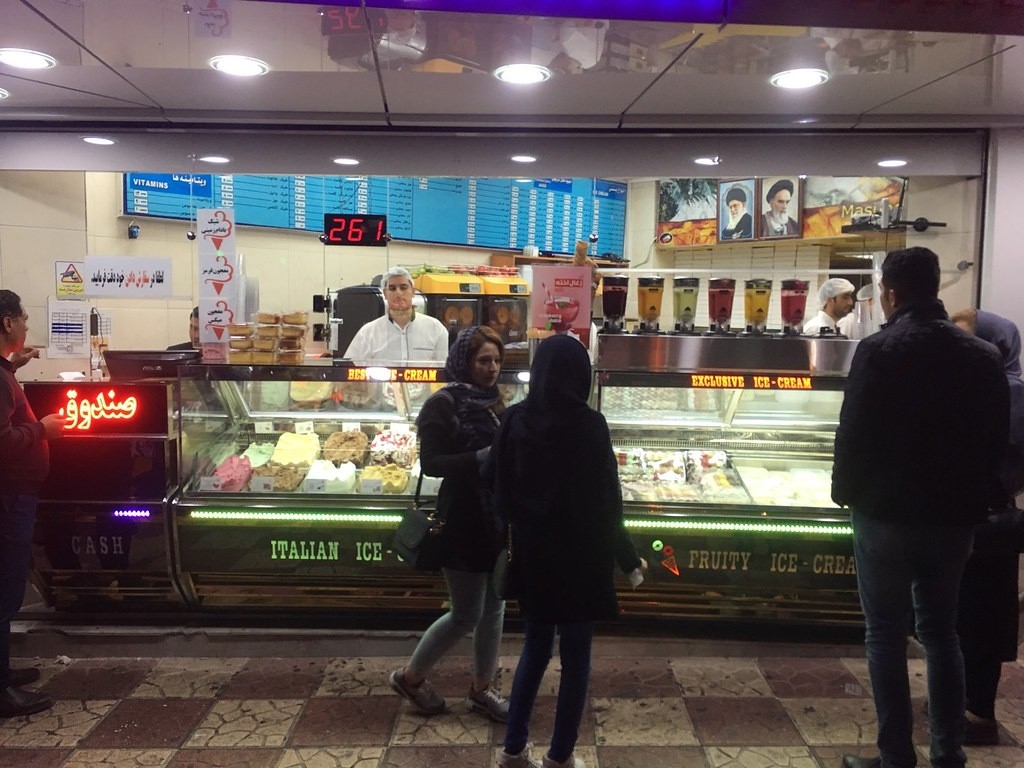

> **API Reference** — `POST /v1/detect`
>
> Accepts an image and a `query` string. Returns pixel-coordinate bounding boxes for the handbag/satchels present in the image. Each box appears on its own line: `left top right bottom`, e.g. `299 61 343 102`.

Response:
391 506 441 571
491 549 521 599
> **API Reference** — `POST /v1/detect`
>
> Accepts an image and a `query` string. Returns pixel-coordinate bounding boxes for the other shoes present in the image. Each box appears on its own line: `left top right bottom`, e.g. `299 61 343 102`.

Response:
542 750 586 768
498 743 540 768
960 709 1000 747
922 699 929 712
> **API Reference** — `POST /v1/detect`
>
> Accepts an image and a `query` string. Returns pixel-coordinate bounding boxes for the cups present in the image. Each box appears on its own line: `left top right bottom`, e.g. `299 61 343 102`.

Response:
851 301 870 339
871 252 887 335
523 246 538 257
544 296 579 334
575 240 589 266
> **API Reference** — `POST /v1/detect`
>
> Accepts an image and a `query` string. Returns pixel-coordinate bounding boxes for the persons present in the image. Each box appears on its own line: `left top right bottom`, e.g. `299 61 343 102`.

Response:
801 277 875 339
493 335 649 768
164 306 201 349
923 308 1024 748
339 266 450 412
389 324 510 726
0 289 66 715
832 248 1015 768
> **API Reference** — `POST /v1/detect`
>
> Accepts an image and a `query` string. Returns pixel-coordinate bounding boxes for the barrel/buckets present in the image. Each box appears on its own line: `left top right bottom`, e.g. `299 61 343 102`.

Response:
856 281 872 320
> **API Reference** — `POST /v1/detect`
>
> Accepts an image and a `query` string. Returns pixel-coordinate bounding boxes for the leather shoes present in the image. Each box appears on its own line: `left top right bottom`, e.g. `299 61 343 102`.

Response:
842 754 882 768
0 683 52 717
0 667 41 688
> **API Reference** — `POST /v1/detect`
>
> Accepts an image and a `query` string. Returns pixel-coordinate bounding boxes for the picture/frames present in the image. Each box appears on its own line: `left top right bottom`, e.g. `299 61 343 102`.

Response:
716 176 759 244
757 175 804 240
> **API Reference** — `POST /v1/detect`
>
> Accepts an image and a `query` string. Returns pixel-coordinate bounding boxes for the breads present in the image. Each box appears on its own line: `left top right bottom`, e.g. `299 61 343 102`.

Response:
229 313 307 365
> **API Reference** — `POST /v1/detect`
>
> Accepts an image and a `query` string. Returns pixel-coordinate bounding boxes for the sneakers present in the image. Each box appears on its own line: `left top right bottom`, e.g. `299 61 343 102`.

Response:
464 682 510 722
388 665 446 711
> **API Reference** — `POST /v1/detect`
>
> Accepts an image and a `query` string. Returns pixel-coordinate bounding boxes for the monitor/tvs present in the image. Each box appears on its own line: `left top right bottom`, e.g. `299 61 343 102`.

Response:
103 350 201 382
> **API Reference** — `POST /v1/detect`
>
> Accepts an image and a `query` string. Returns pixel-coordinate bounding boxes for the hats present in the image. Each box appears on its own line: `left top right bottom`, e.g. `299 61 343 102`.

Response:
726 188 747 204
766 180 794 203
820 278 856 308
